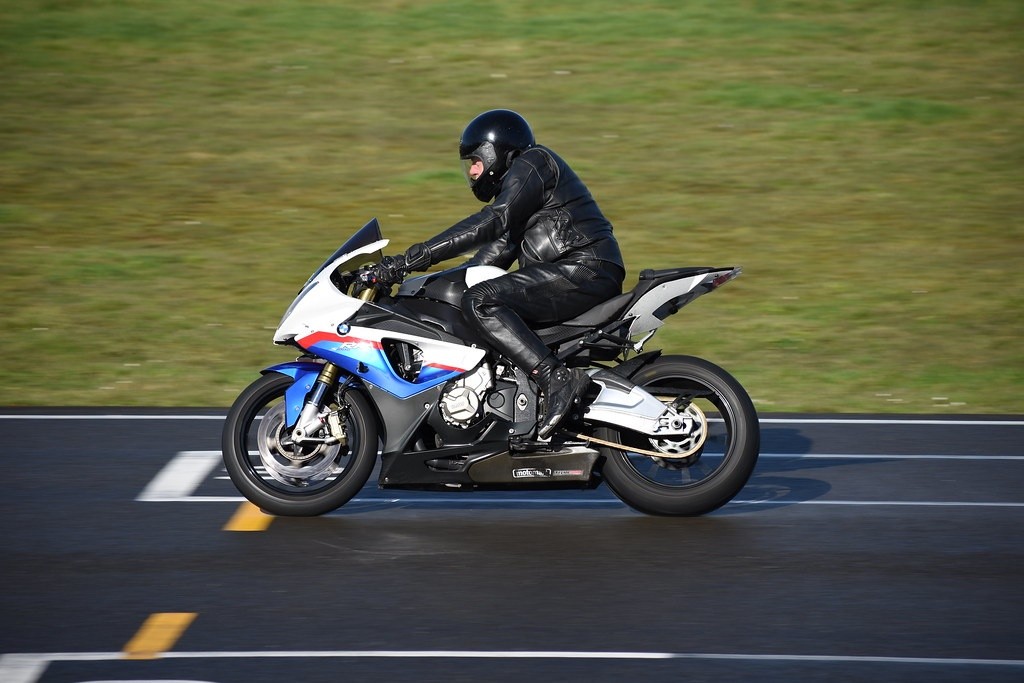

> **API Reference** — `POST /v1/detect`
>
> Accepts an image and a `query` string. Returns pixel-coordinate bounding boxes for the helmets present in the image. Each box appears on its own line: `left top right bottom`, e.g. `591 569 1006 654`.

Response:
460 109 535 203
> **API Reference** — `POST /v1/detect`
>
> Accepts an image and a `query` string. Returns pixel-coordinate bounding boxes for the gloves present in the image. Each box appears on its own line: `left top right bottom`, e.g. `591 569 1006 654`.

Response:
374 254 408 288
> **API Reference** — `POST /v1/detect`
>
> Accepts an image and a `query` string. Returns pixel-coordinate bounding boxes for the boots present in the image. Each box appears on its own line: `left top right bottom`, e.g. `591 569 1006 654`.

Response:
527 352 592 440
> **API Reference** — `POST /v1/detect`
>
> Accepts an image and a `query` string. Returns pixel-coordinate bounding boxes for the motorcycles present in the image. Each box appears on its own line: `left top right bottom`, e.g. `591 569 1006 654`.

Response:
221 216 762 518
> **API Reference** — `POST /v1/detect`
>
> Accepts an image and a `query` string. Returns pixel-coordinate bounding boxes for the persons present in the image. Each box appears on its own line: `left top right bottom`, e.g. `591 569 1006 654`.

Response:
375 109 627 438
